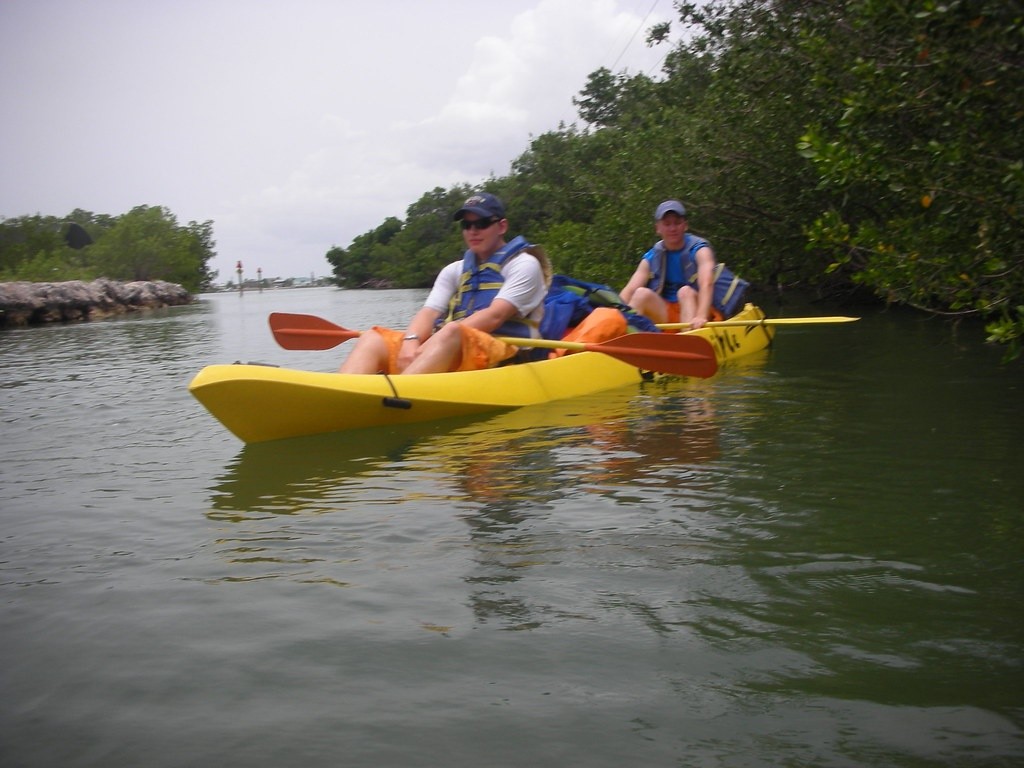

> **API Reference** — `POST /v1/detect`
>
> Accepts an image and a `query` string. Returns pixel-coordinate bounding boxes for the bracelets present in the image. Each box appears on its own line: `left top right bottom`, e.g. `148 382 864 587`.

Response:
401 332 420 341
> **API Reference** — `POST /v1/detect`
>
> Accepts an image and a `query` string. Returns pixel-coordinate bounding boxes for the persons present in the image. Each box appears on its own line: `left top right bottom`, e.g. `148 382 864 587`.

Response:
336 191 552 374
617 200 716 334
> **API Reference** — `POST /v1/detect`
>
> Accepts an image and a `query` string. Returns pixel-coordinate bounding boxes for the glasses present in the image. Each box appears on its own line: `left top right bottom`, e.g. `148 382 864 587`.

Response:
460 217 500 230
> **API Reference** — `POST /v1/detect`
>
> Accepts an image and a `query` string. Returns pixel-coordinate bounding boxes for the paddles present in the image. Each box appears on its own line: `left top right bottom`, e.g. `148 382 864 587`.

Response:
267 310 718 379
651 315 862 331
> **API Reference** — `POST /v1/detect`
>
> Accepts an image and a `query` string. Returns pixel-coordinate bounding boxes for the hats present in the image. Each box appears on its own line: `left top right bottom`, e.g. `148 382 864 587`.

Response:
655 200 687 220
454 193 504 221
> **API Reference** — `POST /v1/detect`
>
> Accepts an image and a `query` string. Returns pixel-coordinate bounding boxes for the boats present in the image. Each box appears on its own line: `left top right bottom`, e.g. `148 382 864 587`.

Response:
186 301 777 443
